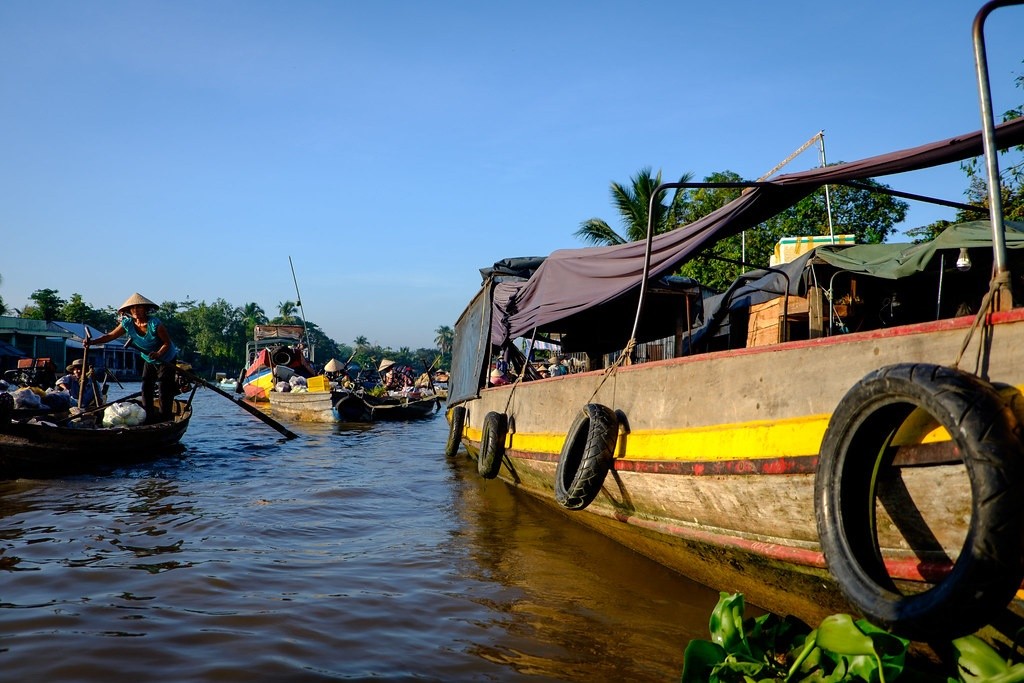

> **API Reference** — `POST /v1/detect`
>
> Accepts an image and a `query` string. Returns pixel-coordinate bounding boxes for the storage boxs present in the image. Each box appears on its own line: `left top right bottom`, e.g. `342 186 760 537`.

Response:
745 296 807 348
769 234 856 267
306 374 330 392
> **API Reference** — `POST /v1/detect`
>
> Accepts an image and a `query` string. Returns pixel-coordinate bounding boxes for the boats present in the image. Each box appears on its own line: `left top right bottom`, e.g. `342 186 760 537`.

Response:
264 384 349 423
440 1 1023 633
235 325 317 402
0 340 194 456
327 388 439 423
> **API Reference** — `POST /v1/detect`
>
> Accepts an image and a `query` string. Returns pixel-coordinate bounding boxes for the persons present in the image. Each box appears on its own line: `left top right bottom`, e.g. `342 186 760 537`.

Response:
55 359 104 421
84 292 178 426
324 358 345 389
537 355 572 380
414 366 450 389
490 368 512 386
378 359 404 390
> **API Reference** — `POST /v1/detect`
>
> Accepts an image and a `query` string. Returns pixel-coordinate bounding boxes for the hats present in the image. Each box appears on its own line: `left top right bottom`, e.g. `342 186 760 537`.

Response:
67 359 91 371
117 293 160 314
324 357 345 372
548 357 559 364
377 359 396 372
537 365 547 371
436 369 444 373
490 368 504 376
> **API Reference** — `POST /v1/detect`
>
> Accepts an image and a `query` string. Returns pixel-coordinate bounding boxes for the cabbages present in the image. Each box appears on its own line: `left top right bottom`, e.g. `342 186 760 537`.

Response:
102 404 146 427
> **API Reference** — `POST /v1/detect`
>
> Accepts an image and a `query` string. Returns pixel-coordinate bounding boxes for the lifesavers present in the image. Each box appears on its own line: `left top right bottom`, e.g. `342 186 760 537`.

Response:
554 403 619 512
812 361 1024 644
271 346 294 366
477 410 506 480
445 406 466 457
